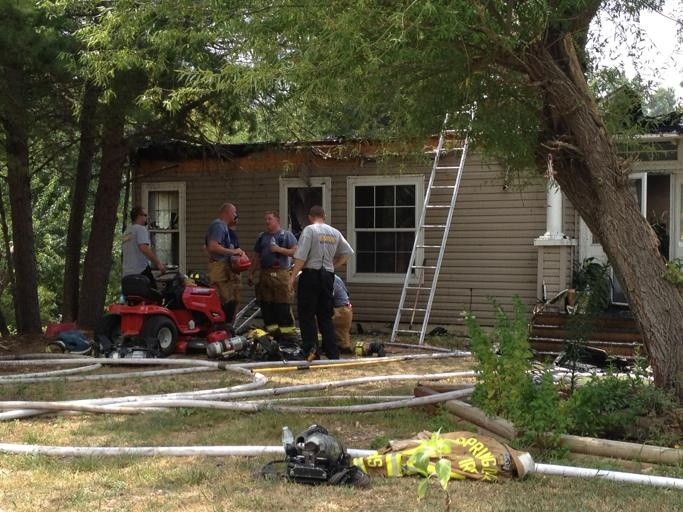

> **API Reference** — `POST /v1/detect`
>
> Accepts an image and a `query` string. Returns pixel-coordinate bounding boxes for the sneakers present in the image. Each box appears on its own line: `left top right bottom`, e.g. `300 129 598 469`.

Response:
370 342 385 357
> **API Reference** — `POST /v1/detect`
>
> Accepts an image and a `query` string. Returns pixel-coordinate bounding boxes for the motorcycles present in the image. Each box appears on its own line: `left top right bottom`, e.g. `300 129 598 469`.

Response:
95 265 237 357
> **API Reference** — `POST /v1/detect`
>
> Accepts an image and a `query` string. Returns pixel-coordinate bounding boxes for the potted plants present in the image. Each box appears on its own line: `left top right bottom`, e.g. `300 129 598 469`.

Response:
566 256 613 314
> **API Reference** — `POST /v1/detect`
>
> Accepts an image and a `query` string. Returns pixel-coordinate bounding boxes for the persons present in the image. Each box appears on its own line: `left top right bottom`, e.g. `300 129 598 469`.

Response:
121 206 166 289
201 202 242 311
294 270 385 357
228 212 241 311
292 204 355 360
247 210 296 327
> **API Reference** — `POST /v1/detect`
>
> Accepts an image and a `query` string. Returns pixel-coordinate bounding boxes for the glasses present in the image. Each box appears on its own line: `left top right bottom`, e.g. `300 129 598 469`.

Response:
140 214 147 216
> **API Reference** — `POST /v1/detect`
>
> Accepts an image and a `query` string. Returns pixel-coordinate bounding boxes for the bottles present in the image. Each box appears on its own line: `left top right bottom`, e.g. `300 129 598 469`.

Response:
281 425 295 450
270 235 274 246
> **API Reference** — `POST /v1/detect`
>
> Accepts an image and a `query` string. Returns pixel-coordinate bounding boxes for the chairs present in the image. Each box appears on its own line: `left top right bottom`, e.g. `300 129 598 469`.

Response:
122 274 164 302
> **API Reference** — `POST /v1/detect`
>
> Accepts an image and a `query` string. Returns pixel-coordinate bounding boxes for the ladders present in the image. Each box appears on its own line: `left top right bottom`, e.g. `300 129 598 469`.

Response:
388 103 475 346
233 261 302 334
